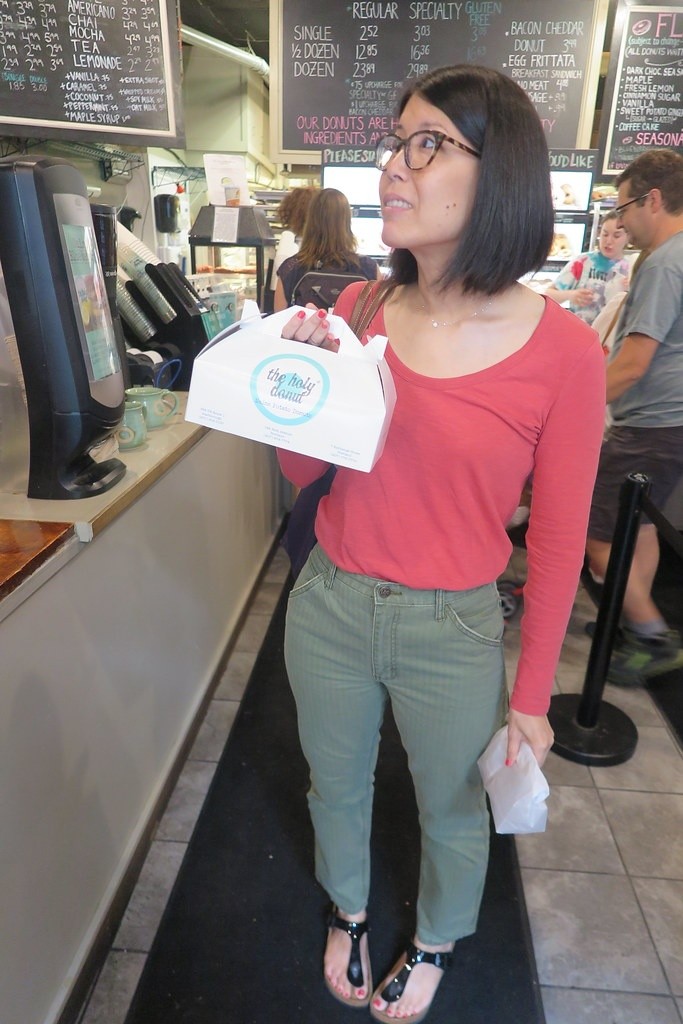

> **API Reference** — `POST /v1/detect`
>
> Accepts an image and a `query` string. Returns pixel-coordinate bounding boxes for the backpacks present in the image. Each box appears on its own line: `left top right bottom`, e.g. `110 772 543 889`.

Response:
290 254 377 311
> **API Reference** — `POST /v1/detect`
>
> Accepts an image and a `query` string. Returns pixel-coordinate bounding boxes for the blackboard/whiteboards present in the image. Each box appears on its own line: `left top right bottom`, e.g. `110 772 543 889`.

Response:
270 0 609 165
1 0 187 151
594 1 683 182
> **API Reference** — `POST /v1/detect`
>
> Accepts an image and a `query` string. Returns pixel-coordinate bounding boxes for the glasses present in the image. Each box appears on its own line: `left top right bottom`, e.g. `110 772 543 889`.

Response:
614 193 649 218
373 132 482 171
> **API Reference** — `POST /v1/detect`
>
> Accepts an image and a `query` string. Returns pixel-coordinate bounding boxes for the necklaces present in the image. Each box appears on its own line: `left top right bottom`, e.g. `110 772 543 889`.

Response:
412 291 494 329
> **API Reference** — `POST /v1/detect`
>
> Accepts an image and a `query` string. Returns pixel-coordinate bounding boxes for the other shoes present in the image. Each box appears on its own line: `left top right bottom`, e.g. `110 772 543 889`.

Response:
506 506 530 530
585 616 683 686
589 566 605 583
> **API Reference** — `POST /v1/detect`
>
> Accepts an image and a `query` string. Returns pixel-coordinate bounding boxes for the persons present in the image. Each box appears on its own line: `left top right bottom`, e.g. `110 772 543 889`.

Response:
270 185 384 314
543 149 683 687
275 64 609 1024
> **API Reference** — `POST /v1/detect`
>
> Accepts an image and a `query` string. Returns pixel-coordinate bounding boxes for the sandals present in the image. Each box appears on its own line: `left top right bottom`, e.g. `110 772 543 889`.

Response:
322 905 372 1010
370 933 455 1023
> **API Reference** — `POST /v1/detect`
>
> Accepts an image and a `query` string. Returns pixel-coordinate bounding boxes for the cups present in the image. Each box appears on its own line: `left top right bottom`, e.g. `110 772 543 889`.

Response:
114 387 179 449
115 220 208 344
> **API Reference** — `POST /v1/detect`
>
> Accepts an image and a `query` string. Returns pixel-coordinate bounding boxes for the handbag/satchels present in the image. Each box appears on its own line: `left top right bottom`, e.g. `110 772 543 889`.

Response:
277 280 403 579
